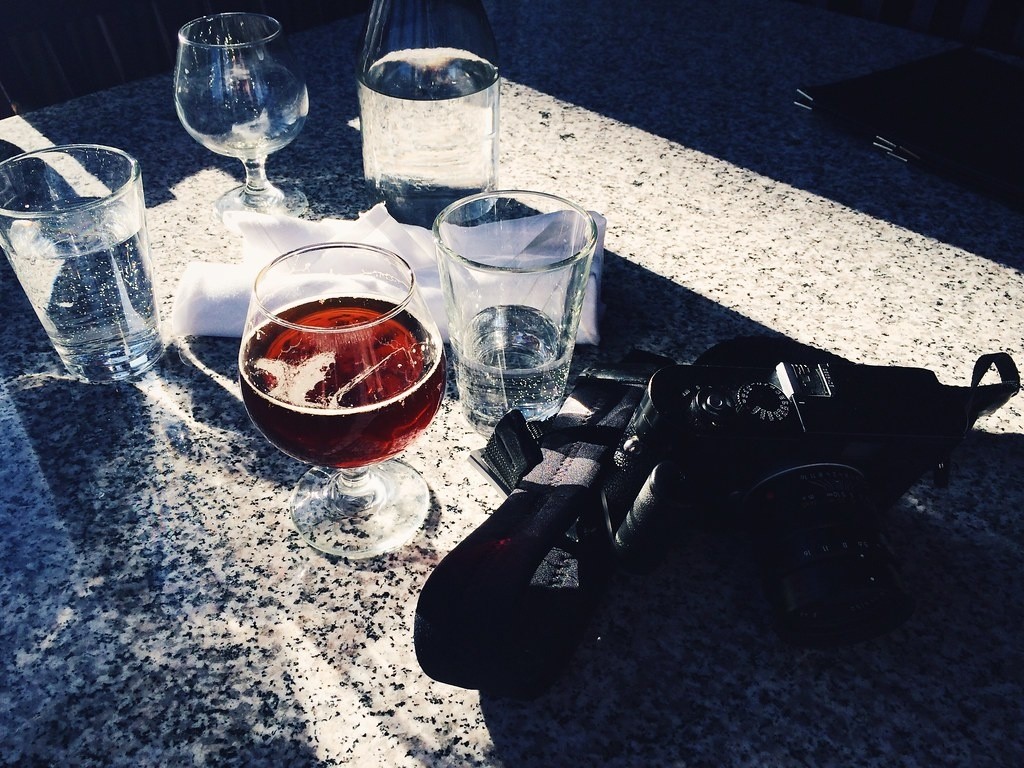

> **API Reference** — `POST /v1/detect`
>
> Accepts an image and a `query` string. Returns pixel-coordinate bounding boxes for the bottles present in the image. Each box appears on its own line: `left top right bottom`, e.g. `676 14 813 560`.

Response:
356 1 500 229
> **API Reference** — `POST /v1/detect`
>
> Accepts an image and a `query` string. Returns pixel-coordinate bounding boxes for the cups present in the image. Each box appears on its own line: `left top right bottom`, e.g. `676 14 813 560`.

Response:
1 144 165 385
434 191 598 436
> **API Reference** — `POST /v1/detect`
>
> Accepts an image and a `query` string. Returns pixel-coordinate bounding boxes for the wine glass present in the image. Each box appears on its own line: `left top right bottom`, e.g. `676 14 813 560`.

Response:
239 241 446 562
172 15 310 220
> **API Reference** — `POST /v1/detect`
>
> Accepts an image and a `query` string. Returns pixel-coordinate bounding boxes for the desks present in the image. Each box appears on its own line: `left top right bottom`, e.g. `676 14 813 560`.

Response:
0 0 1024 768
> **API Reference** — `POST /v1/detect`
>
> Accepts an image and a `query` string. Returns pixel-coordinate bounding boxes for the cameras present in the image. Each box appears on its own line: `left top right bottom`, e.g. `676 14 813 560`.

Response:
613 365 947 650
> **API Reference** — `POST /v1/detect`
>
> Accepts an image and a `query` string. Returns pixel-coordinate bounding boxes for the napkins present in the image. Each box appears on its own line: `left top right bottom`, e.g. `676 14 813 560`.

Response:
169 197 608 346
